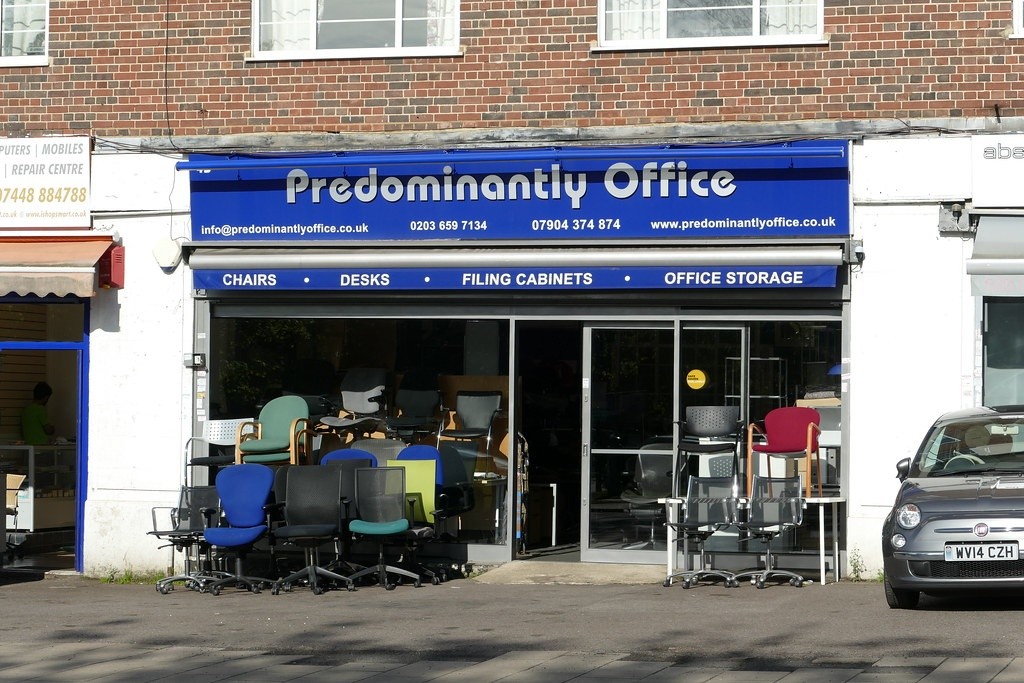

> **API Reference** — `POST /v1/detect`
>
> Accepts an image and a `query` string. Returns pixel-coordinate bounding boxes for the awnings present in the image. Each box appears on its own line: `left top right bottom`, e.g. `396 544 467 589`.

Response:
0 239 113 298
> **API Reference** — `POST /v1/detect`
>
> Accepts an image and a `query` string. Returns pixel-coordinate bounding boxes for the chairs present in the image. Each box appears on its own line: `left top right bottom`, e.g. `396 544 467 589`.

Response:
145 373 507 596
619 406 823 589
927 434 1013 477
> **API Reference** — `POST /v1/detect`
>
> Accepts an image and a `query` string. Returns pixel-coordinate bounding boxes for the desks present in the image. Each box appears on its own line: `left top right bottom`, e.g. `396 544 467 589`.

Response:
658 498 846 586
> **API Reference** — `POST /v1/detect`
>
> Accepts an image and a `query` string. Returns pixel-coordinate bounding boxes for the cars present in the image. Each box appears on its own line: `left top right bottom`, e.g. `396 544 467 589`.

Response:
881 405 1024 610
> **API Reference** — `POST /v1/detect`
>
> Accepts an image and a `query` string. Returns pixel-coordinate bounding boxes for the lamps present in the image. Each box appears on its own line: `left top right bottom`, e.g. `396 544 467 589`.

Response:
951 204 962 221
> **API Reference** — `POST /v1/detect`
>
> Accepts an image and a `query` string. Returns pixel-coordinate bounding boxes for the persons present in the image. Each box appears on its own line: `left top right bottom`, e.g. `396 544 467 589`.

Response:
21 381 56 479
